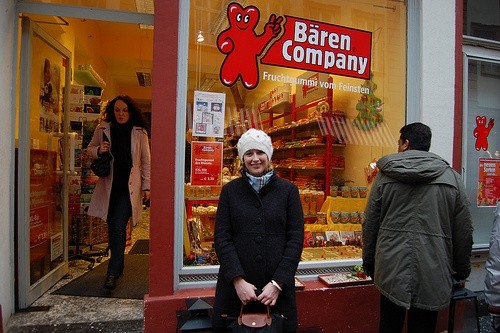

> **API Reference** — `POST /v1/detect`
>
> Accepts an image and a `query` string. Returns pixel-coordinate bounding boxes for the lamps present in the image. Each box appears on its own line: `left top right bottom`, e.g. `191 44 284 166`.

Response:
211 0 246 35
433 284 480 333
136 0 155 29
175 297 214 333
198 74 219 91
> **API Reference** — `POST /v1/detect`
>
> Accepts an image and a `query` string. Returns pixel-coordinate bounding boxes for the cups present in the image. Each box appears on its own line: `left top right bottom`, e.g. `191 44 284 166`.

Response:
351 212 359 224
330 211 341 224
330 186 338 197
316 212 327 224
360 212 364 224
359 187 369 198
350 186 359 198
341 186 350 198
341 212 351 223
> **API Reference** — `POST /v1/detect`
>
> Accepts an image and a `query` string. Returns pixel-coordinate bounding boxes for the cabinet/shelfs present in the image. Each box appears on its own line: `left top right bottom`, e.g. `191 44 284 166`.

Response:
184 76 367 260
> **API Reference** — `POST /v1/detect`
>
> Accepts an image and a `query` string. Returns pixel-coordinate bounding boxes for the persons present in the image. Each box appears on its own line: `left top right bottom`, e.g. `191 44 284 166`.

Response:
185 129 216 184
85 96 151 290
214 128 305 333
40 59 59 113
361 122 474 333
484 200 500 333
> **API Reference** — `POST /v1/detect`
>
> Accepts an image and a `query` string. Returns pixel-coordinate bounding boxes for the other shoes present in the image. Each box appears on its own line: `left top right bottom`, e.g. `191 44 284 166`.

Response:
101 266 122 293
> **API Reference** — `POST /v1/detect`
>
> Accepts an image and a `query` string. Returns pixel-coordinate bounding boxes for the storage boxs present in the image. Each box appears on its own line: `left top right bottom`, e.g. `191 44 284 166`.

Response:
70 83 110 247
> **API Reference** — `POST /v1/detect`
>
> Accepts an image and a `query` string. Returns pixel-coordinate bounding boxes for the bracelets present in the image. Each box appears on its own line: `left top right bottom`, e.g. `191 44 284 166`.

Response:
271 279 283 291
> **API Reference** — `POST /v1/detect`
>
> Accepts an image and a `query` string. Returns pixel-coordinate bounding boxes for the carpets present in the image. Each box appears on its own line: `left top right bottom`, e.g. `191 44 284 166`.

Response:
128 239 150 254
47 254 149 300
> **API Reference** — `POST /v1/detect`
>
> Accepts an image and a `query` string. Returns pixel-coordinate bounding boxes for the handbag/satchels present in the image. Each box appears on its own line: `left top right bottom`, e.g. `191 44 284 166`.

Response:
220 293 289 333
90 134 112 179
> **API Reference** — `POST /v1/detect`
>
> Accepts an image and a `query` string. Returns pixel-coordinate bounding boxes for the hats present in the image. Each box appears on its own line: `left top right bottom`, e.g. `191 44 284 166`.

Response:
237 127 273 165
